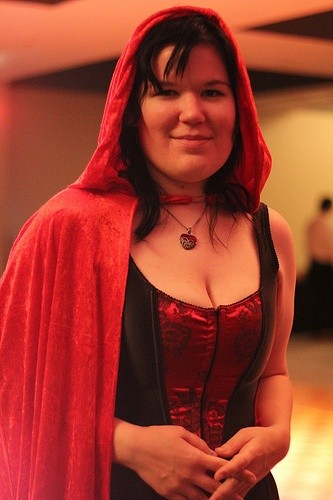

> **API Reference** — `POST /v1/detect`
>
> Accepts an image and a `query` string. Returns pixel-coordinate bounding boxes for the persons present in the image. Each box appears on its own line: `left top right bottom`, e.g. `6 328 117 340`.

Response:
0 7 300 500
307 195 333 342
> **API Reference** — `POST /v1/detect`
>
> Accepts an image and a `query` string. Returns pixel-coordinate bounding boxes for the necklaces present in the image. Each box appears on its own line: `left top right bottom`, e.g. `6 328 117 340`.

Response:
159 198 208 250
